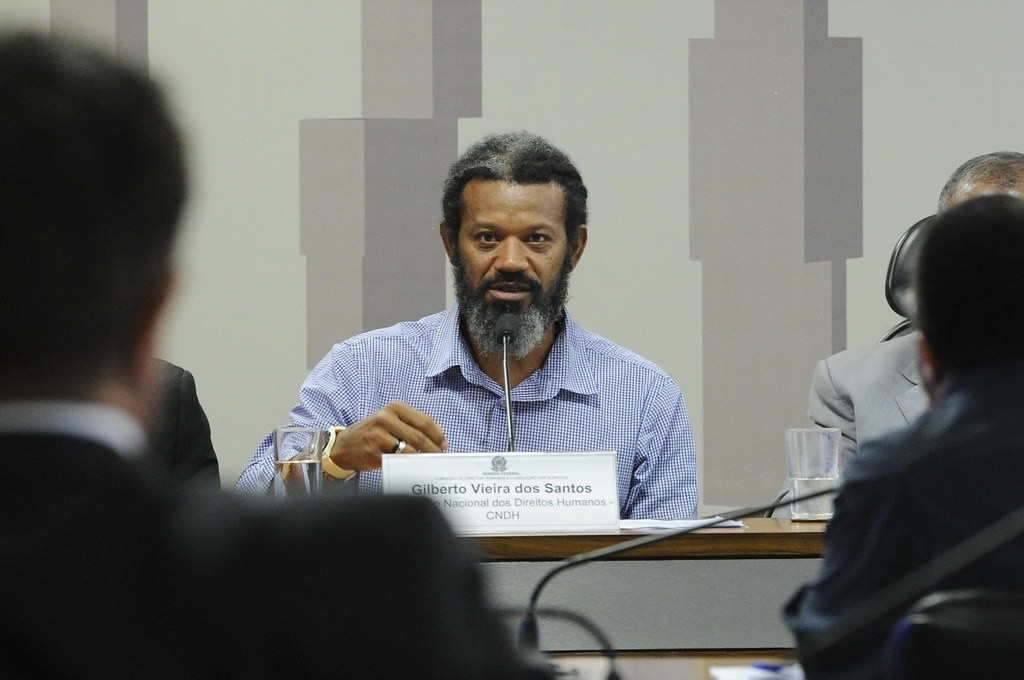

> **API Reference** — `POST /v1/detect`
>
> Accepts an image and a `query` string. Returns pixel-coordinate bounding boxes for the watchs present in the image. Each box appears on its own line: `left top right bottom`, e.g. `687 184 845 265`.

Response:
313 423 359 482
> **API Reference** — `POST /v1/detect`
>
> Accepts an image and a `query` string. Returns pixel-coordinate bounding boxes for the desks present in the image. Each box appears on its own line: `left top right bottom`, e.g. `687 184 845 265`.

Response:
460 518 827 680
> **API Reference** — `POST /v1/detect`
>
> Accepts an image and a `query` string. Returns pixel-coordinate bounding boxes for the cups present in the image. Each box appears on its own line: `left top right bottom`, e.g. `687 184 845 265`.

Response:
270 427 323 502
786 428 840 522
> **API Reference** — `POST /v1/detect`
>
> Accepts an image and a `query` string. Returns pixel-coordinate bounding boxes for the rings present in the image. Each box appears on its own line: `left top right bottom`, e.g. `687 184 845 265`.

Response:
392 437 407 456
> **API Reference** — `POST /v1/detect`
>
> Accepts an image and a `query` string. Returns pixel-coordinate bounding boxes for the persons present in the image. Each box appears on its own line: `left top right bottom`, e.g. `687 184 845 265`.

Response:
767 150 1024 518
779 193 1024 680
126 348 221 488
233 128 698 522
0 24 550 680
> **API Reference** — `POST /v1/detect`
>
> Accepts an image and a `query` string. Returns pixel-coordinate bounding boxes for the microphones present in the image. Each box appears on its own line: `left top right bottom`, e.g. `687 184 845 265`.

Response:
494 314 521 452
